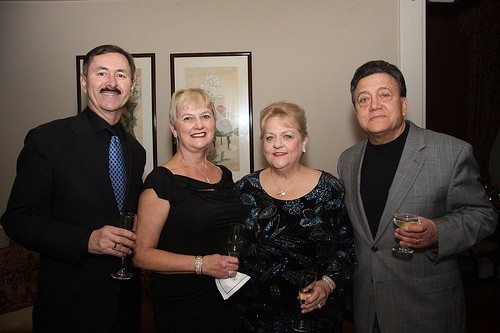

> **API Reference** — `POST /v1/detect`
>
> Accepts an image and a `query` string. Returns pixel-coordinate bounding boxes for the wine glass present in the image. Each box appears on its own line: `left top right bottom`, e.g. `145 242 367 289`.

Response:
111 212 138 280
291 270 318 332
392 212 422 253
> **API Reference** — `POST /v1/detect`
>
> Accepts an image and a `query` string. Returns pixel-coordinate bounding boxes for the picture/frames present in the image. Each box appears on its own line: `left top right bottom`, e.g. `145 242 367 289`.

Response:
75 53 157 182
170 51 254 182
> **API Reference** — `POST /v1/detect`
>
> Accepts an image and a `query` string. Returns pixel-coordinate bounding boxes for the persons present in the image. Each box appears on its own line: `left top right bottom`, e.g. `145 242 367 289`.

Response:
0 45 147 333
235 102 356 332
336 59 497 333
132 88 239 332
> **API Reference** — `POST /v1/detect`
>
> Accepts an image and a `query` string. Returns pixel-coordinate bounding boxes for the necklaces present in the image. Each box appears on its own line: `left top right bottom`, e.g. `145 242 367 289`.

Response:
271 164 301 197
174 155 211 183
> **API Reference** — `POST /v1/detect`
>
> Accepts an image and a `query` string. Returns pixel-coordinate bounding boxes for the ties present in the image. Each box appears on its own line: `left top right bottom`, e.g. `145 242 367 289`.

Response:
108 135 127 214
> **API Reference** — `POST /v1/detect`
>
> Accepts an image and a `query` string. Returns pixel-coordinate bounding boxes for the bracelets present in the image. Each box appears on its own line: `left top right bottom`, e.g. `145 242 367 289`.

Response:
194 256 203 275
322 276 333 293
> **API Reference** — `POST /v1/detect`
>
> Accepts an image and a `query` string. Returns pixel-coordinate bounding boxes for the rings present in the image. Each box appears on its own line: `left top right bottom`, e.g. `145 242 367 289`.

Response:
318 303 321 308
228 270 232 277
112 243 116 250
416 239 418 244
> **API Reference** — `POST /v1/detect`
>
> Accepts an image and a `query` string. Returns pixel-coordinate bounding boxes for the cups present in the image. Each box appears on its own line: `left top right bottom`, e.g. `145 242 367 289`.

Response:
226 223 244 257
